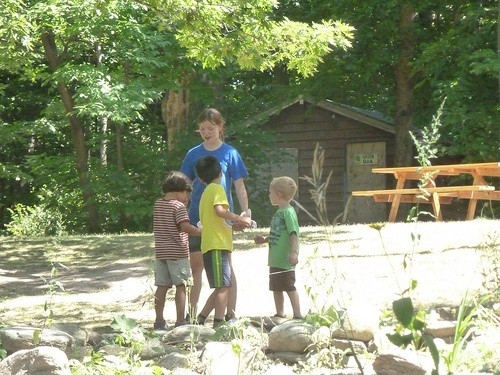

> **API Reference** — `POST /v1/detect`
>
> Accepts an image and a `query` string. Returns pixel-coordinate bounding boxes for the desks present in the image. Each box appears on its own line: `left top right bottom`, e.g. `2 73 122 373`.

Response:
372 162 500 222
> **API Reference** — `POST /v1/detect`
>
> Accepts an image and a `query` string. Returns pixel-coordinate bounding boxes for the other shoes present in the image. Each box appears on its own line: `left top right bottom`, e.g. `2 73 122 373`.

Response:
154 320 165 330
213 320 227 330
174 322 189 326
263 315 287 326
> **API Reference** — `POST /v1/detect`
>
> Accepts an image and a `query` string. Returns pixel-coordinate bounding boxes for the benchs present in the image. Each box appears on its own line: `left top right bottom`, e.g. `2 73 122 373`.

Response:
352 185 495 221
451 190 500 221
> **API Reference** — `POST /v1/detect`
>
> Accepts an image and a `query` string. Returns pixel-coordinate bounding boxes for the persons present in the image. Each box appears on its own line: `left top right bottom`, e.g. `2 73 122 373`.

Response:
254 176 306 318
181 110 250 323
195 156 251 324
151 171 192 328
